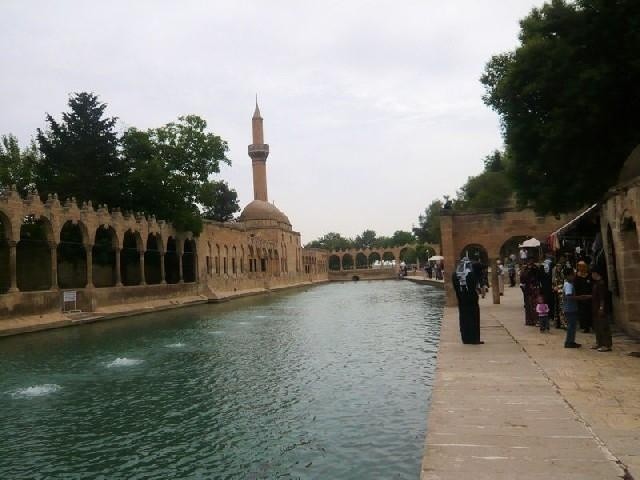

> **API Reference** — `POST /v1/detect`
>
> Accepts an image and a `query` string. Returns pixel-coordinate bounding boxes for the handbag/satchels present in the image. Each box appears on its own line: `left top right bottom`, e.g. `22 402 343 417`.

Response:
565 298 578 313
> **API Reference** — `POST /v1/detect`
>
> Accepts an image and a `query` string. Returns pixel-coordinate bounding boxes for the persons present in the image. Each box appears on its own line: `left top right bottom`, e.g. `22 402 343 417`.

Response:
518 240 609 334
580 265 613 351
562 267 587 348
424 262 444 281
468 246 518 300
451 256 485 344
404 264 408 276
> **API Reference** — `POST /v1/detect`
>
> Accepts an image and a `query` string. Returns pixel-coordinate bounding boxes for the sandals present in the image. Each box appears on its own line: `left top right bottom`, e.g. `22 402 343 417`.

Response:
589 343 612 352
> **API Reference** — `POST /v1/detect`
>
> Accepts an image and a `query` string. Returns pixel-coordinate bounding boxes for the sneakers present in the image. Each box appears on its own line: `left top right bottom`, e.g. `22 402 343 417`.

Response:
564 342 581 348
